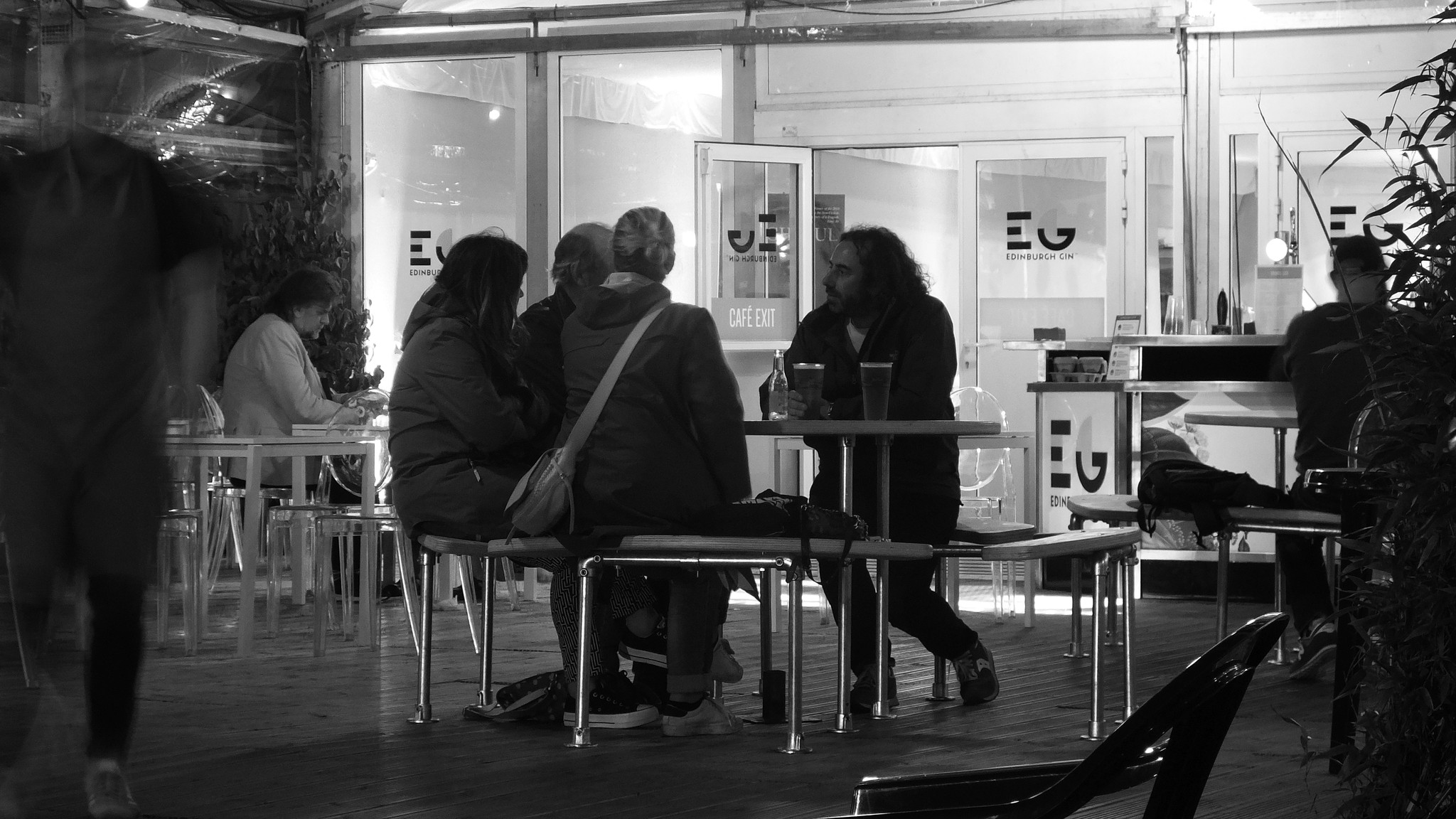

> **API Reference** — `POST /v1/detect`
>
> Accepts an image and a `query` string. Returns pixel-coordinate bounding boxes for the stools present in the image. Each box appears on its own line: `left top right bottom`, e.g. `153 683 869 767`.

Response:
156 478 406 659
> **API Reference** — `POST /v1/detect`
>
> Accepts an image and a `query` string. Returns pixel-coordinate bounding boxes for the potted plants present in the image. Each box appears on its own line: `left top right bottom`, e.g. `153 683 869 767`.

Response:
214 148 396 598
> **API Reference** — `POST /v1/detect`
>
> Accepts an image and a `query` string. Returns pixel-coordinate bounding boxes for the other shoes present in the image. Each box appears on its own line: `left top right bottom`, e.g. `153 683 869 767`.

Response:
85 760 142 818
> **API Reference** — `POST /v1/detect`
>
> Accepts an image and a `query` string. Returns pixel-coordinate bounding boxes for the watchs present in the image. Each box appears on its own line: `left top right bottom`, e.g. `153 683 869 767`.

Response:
828 401 836 418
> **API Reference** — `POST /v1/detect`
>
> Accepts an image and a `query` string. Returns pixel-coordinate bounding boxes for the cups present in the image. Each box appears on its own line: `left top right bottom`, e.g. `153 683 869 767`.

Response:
1163 295 1185 335
859 362 895 421
792 363 826 421
1190 320 1207 335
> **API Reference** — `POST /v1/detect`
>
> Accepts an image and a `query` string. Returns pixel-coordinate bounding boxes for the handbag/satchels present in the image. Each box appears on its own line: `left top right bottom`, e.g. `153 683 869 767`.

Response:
799 504 869 585
1138 459 1284 536
503 446 577 546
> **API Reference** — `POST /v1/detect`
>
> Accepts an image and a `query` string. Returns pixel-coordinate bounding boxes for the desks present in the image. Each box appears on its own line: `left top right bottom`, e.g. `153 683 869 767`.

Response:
167 416 390 660
745 421 1001 733
1184 414 1300 664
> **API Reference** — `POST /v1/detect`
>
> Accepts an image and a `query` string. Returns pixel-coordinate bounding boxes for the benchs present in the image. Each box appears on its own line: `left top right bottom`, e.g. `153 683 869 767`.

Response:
407 493 1341 747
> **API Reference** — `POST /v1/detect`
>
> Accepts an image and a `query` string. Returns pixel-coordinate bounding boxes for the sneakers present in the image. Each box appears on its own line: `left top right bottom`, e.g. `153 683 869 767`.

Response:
663 695 744 737
850 664 900 714
948 638 1000 706
710 634 744 683
564 670 658 729
1288 620 1338 680
618 614 667 669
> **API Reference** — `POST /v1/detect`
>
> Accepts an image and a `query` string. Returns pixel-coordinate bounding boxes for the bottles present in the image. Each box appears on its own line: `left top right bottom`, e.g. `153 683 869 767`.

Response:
1244 306 1257 335
768 349 789 420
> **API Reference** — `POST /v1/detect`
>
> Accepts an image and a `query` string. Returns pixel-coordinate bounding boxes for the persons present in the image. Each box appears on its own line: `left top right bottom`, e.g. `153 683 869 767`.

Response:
0 37 225 819
760 227 1001 718
221 269 392 604
1274 235 1397 681
384 208 752 739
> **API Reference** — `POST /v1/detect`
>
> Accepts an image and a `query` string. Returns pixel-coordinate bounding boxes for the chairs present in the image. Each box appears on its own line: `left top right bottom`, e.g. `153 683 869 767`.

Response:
818 612 1291 819
1305 391 1408 776
